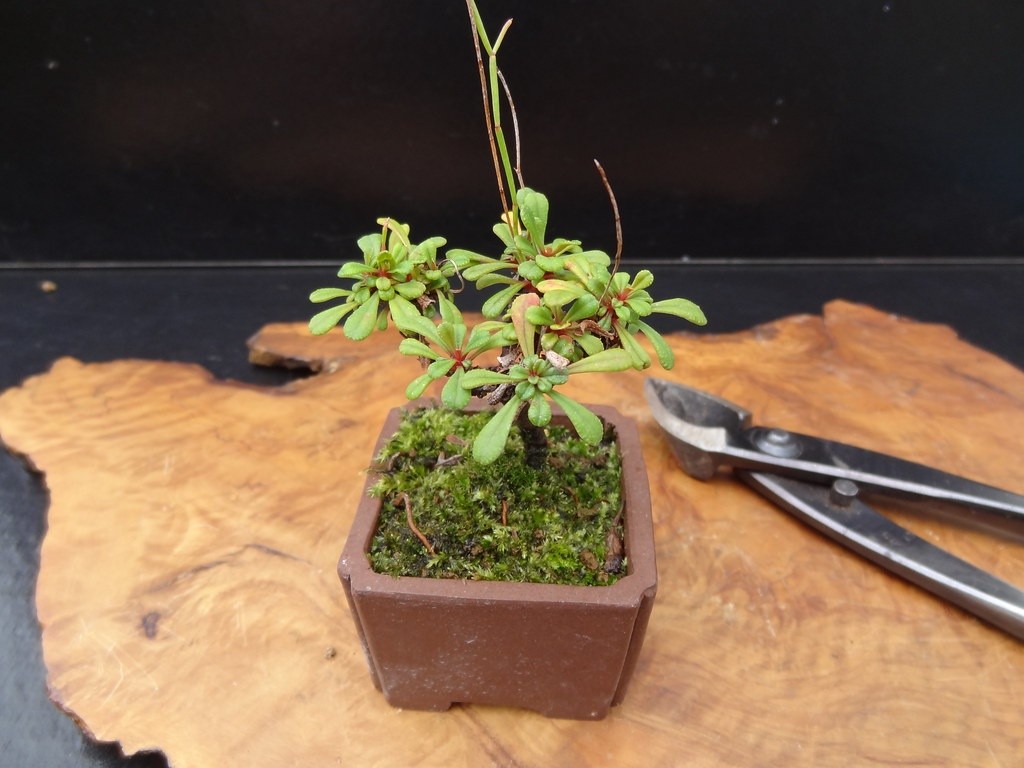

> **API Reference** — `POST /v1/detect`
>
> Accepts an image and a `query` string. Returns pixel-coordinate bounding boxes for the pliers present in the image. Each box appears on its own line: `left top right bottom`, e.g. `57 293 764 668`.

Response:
643 372 1024 644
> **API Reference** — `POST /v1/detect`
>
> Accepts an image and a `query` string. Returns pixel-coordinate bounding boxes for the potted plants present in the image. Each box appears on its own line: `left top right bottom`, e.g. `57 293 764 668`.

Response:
307 0 709 720
